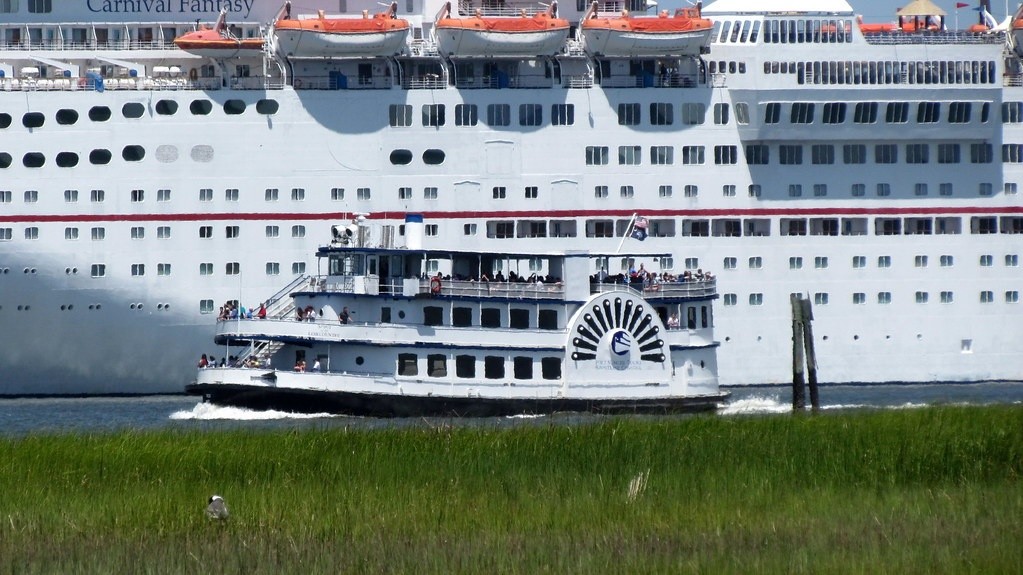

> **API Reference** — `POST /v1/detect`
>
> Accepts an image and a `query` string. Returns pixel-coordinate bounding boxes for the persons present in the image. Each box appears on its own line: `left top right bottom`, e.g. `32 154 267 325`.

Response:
339 305 353 325
312 358 321 372
217 299 246 320
209 355 218 368
666 312 679 331
589 263 714 284
294 306 305 322
256 303 267 318
659 64 668 86
247 307 254 319
220 352 272 368
198 353 209 368
305 307 317 323
414 270 562 283
295 360 306 373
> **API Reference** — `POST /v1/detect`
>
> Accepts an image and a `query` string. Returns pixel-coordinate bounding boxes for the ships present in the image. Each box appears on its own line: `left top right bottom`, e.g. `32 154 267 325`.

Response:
0 0 1023 401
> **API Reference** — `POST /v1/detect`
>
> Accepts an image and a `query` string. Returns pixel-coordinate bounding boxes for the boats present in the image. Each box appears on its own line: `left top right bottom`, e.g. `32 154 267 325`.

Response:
578 0 715 59
169 7 268 60
269 1 410 59
179 206 732 412
429 0 573 57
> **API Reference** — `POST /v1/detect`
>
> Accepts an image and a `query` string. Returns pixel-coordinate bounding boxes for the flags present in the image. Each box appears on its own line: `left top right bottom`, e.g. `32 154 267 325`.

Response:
634 215 648 229
629 226 649 241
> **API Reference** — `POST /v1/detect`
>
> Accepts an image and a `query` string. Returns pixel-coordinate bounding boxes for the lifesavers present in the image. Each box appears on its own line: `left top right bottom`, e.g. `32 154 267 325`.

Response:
431 277 442 292
